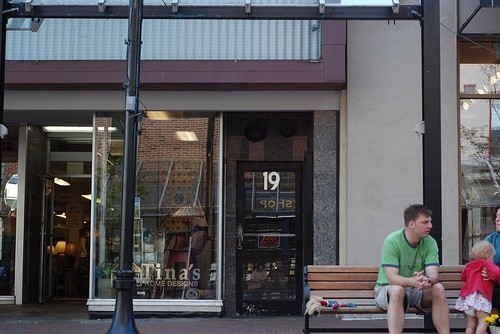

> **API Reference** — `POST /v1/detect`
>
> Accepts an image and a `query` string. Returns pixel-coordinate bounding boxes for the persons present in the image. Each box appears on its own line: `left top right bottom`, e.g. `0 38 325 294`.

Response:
456 240 500 334
250 258 290 299
374 204 451 334
480 206 500 334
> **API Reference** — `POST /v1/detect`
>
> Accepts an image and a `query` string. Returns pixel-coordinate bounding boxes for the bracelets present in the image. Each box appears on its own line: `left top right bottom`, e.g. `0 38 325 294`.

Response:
426 275 431 284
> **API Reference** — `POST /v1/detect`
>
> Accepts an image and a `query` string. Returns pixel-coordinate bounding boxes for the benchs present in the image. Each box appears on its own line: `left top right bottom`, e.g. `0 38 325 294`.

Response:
301 265 466 334
